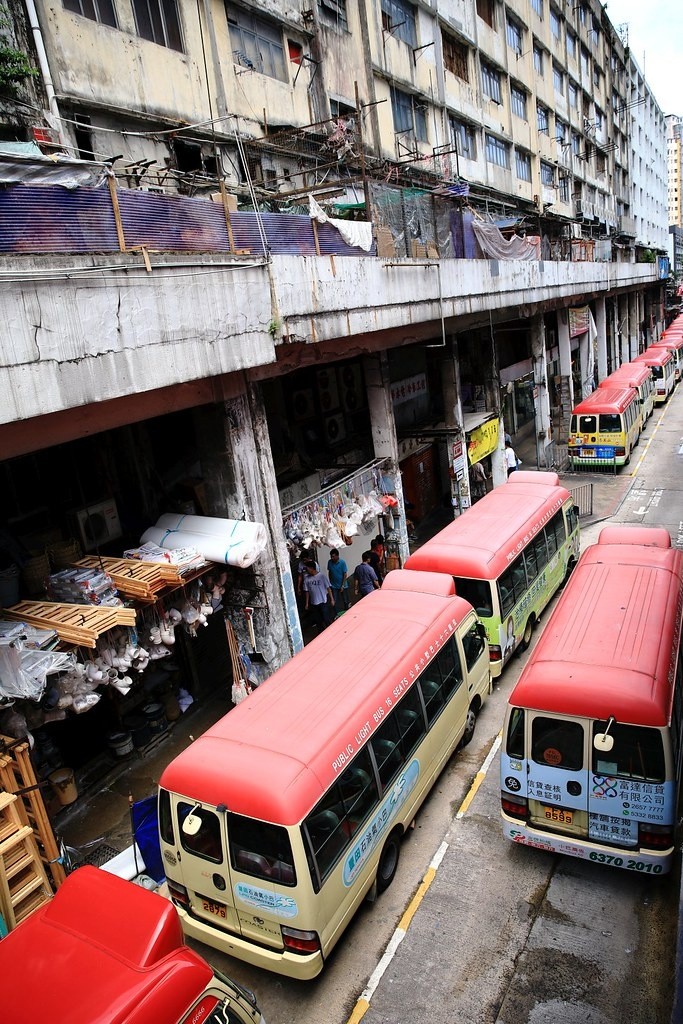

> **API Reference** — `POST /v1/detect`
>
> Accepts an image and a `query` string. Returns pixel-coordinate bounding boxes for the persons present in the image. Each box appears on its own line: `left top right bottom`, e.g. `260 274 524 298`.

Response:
505 431 512 445
327 549 348 613
352 551 381 597
375 535 386 566
405 518 418 543
505 441 518 475
365 538 383 586
303 561 335 631
296 552 319 595
472 463 488 497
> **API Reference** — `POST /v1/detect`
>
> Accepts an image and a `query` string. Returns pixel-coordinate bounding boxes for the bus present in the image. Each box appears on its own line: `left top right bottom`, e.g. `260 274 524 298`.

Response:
155 569 491 984
567 311 683 470
1 864 277 1024
499 523 683 882
410 470 583 688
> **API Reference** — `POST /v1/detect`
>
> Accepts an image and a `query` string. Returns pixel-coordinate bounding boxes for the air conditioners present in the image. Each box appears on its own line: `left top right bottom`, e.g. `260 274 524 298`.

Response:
76 497 126 553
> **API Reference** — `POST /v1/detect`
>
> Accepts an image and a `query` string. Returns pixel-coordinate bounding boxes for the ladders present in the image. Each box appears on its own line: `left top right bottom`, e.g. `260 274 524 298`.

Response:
70 554 183 604
0 734 67 934
4 599 138 639
5 605 136 649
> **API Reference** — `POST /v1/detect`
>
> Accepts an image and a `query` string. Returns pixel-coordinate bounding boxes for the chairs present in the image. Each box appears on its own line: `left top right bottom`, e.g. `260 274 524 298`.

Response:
571 427 622 434
234 638 479 885
474 523 566 622
